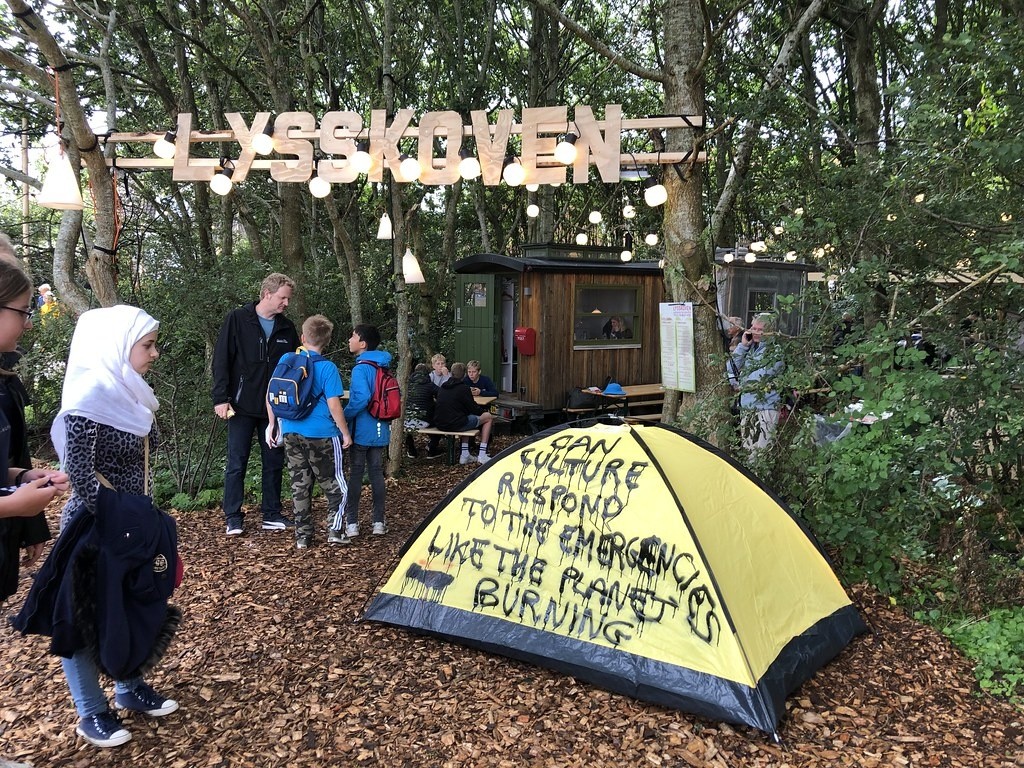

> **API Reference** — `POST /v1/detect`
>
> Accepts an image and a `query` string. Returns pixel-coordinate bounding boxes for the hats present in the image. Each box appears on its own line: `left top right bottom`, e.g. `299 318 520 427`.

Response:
603 383 626 395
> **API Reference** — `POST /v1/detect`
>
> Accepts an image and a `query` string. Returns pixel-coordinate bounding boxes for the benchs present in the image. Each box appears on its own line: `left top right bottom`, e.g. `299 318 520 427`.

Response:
562 399 682 422
389 427 480 467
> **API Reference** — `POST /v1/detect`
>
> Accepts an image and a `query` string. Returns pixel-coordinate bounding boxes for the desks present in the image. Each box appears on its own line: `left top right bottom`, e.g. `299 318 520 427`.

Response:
340 390 498 451
581 383 665 417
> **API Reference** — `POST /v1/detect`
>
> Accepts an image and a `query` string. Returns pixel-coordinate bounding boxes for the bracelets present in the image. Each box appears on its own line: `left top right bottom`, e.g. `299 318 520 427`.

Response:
14 470 29 488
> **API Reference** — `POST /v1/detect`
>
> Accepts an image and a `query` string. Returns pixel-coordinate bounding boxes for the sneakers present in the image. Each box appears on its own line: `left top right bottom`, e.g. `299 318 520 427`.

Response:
345 522 360 537
226 517 243 535
261 514 294 529
327 529 351 544
373 522 390 534
297 539 312 549
460 455 478 464
478 455 491 464
114 684 179 716
407 447 417 459
427 449 443 459
75 711 132 748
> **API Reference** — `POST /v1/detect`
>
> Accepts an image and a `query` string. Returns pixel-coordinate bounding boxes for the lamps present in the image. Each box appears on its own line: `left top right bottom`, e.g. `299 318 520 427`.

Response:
376 203 395 239
724 207 834 264
38 64 81 210
403 224 425 285
154 130 581 198
528 176 668 260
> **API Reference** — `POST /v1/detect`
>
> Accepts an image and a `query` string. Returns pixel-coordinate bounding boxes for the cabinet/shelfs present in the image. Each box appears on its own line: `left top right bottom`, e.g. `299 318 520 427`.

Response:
501 280 519 392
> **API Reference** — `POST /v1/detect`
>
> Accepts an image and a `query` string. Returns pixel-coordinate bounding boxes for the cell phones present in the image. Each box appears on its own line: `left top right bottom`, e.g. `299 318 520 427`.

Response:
746 333 753 341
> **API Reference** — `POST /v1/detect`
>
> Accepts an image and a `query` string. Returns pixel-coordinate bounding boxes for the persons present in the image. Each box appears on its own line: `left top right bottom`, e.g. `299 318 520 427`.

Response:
343 323 392 537
59 303 180 750
403 352 499 466
211 272 303 534
610 316 633 339
834 312 853 340
264 314 352 549
724 313 786 483
37 282 59 330
0 243 51 602
0 258 68 570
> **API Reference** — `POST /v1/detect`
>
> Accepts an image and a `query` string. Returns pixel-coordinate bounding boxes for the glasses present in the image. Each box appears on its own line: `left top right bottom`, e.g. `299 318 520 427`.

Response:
0 308 35 319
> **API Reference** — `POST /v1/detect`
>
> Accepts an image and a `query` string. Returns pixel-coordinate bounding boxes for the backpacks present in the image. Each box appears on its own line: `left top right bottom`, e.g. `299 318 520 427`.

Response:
268 355 328 421
355 360 401 422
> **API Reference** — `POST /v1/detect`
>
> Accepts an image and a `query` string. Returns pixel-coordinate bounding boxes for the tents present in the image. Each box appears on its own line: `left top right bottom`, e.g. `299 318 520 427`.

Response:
351 413 881 747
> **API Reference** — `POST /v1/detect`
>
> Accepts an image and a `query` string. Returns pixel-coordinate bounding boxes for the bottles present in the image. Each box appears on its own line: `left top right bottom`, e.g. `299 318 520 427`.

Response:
574 331 587 340
